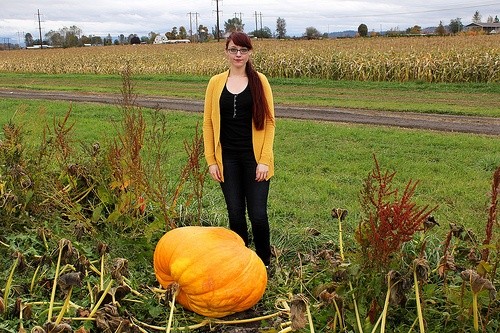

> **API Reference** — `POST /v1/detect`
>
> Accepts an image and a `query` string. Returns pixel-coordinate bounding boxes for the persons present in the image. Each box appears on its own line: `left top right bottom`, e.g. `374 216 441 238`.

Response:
202 31 276 284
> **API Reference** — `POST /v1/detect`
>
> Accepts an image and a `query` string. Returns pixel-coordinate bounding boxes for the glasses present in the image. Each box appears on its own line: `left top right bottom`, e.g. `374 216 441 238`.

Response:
227 48 249 54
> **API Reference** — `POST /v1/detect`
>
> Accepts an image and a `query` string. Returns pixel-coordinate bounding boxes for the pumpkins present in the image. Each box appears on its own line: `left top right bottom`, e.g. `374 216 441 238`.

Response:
153 226 267 316
